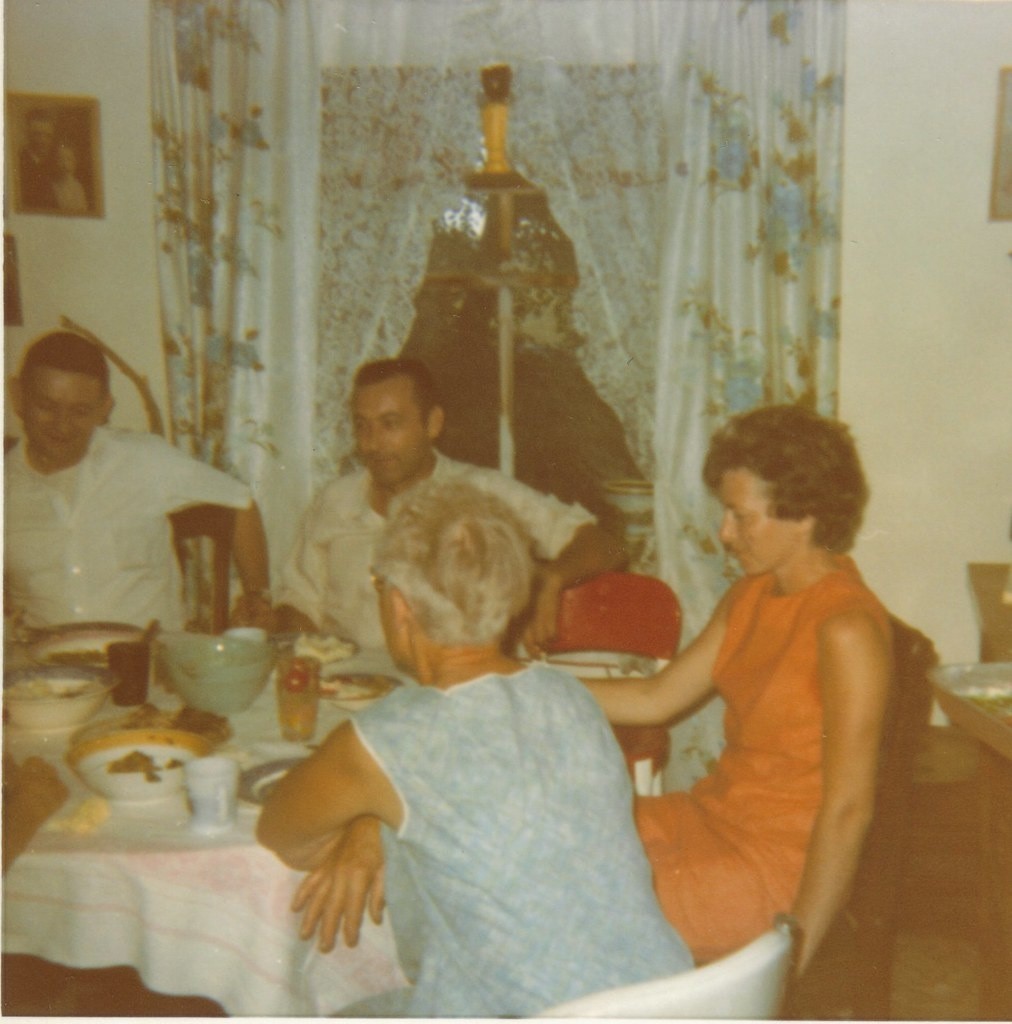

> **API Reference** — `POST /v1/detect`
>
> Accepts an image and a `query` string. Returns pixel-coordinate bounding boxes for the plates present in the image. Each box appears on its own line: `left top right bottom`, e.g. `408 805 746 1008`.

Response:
544 651 670 681
923 660 1011 699
319 673 404 709
271 632 359 669
27 621 145 668
238 756 302 809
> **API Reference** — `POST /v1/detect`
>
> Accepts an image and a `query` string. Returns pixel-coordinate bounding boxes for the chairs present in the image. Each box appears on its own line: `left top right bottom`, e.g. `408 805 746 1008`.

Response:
532 560 1011 1019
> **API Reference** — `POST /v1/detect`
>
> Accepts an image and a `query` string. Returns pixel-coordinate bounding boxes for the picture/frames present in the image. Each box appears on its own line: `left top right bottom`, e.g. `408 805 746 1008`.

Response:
4 91 105 220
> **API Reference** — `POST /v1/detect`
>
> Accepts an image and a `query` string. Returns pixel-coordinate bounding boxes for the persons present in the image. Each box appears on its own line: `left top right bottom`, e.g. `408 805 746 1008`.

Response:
272 357 628 662
575 406 895 978
254 470 697 1018
4 334 274 644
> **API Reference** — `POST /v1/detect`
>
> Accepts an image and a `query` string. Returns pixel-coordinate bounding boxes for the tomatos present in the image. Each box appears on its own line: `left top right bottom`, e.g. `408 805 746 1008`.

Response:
283 657 310 692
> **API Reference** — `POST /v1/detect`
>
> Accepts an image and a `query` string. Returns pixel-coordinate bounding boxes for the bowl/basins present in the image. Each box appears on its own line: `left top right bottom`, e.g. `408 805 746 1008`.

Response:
3 663 115 732
61 729 214 804
163 636 272 718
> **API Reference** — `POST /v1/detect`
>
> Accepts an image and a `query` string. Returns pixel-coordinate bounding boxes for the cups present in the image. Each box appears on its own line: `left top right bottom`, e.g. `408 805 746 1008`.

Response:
108 642 149 707
275 658 320 740
185 756 235 831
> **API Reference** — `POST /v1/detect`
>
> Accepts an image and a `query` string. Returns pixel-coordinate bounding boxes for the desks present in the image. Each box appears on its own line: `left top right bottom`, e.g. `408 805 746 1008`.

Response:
2 623 413 1016
